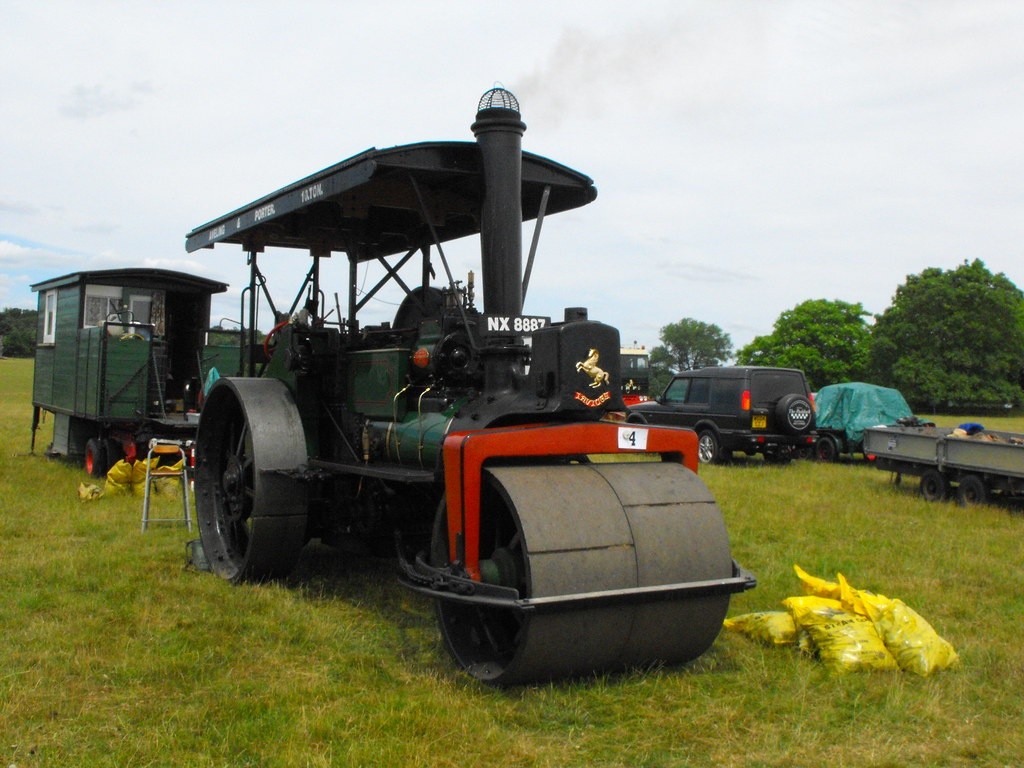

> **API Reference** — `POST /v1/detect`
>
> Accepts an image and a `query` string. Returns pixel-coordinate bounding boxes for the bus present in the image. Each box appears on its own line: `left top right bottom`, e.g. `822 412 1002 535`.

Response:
602 341 656 422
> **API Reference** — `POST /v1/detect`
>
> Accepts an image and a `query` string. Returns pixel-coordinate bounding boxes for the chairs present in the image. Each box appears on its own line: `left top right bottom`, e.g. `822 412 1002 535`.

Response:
142 445 191 533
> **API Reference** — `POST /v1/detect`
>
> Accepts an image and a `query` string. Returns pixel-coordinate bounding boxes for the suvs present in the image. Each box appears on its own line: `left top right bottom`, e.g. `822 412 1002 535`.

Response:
624 364 820 464
